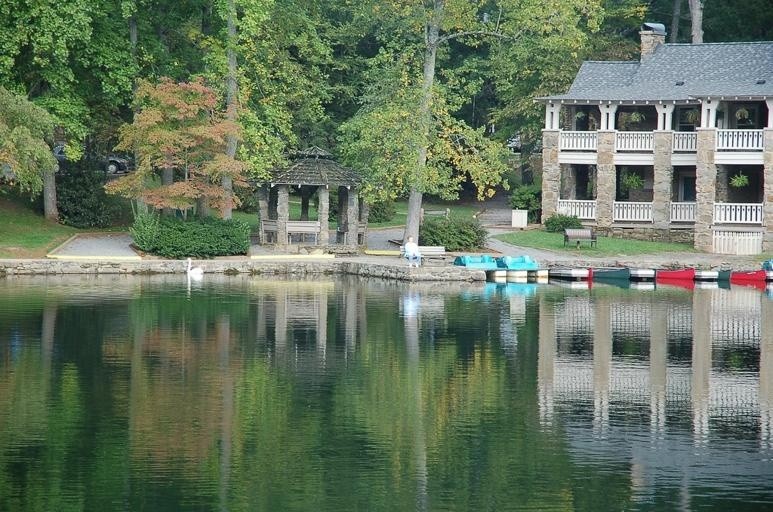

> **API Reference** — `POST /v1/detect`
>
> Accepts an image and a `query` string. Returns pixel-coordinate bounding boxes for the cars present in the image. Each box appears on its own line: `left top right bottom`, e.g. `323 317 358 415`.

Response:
49 143 128 174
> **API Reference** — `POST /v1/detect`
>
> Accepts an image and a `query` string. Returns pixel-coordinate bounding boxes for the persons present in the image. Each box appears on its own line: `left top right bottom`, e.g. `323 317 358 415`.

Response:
403 236 420 268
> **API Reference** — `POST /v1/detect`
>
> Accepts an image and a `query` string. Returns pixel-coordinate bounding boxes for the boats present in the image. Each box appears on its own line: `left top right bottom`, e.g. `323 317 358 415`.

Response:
548 260 773 283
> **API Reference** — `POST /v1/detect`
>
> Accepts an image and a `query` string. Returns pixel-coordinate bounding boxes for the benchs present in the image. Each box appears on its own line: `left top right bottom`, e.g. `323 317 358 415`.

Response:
563 229 597 250
337 221 367 245
399 246 451 268
285 220 321 246
260 219 277 244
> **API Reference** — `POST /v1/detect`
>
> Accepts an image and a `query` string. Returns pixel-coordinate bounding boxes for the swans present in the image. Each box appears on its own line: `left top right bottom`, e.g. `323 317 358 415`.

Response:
185 258 204 274
